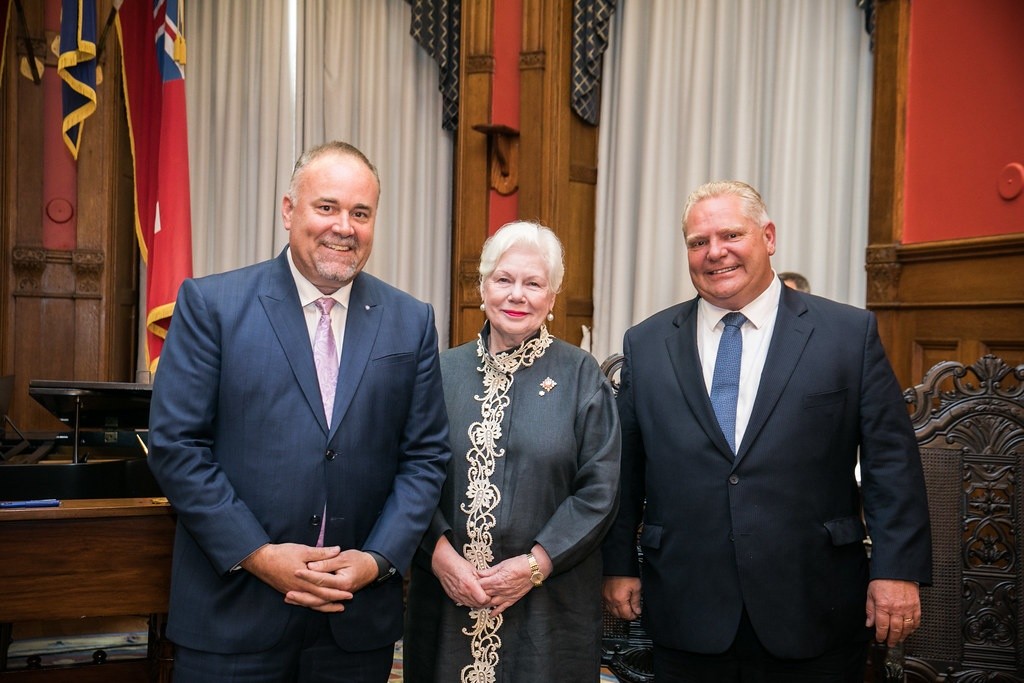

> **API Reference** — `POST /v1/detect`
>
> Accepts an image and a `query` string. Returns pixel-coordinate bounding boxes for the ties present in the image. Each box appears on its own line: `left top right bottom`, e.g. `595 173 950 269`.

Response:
312 297 340 548
709 312 746 456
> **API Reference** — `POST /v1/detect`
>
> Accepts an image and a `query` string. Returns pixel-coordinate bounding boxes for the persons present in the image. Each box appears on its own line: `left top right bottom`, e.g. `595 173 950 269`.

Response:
777 273 808 293
600 181 932 683
403 218 622 683
146 141 453 683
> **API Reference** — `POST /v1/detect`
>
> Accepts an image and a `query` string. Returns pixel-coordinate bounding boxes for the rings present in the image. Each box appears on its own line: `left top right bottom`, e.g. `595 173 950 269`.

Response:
905 619 912 622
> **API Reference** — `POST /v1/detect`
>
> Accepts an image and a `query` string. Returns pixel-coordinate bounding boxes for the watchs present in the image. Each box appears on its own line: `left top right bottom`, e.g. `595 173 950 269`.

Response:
527 553 544 587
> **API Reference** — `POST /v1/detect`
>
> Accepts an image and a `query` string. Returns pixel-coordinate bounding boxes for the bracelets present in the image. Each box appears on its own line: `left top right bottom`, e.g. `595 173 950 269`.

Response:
369 550 396 582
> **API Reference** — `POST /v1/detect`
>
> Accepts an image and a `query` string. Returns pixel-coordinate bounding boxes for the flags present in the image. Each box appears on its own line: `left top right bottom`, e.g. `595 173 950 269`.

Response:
57 0 103 159
115 0 194 371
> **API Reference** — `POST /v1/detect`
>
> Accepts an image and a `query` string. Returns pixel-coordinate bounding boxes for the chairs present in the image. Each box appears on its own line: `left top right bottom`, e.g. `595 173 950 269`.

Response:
861 355 1024 683
599 353 656 682
